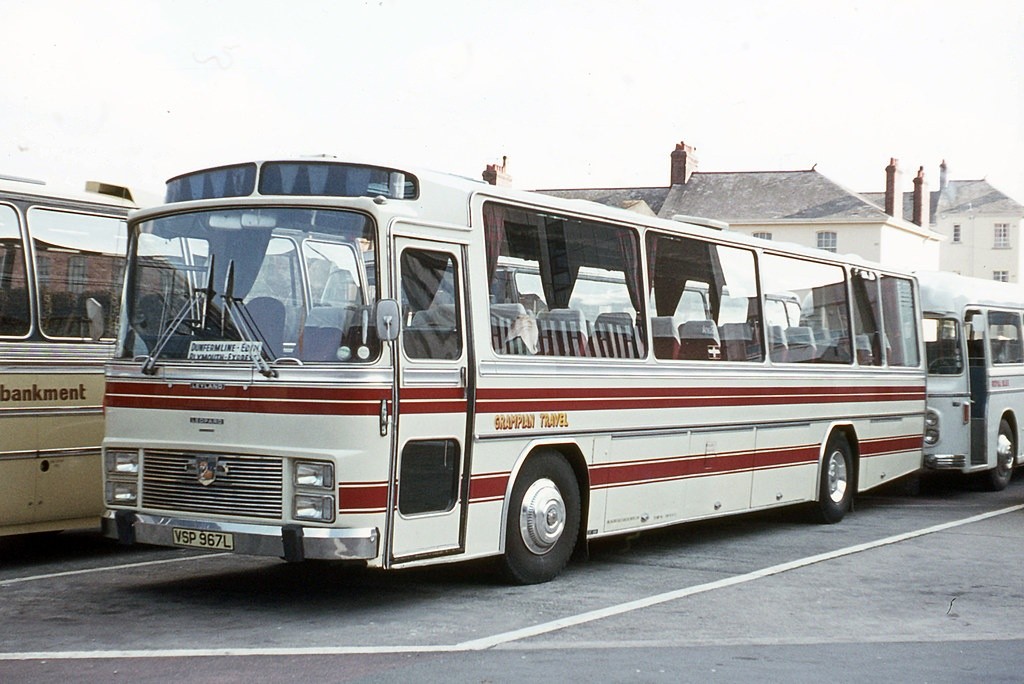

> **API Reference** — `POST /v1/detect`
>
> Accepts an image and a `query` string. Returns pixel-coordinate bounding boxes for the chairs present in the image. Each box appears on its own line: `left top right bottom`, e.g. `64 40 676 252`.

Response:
132 294 895 368
0 281 112 339
929 337 1023 375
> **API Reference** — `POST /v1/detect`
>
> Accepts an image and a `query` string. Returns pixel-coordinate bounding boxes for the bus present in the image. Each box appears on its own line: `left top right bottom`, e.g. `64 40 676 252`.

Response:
85 153 928 590
915 267 1024 492
0 177 213 548
